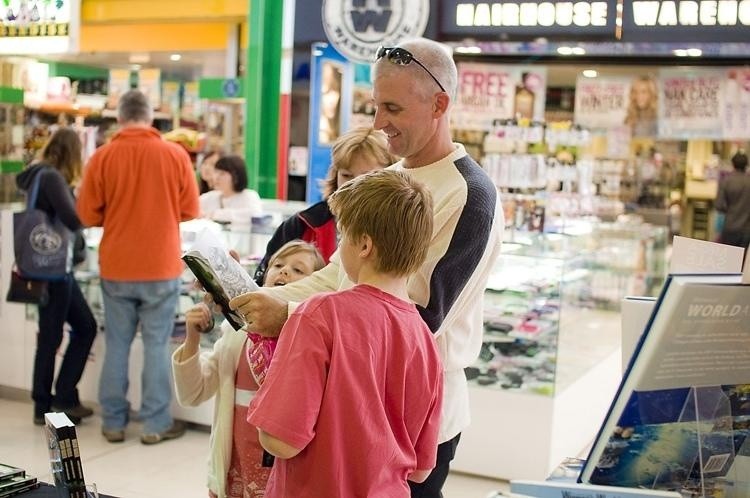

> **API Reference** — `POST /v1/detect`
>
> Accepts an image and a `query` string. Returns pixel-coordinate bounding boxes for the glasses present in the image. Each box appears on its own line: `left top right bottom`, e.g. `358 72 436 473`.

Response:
374 44 446 92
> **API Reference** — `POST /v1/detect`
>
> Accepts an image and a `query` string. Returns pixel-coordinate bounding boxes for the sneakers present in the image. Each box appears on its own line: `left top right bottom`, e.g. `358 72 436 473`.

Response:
141 420 187 444
101 422 126 443
64 404 94 417
34 410 81 426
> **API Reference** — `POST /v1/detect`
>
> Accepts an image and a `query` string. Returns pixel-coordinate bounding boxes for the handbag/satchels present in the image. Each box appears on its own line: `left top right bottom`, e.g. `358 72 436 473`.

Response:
6 272 51 305
13 209 76 282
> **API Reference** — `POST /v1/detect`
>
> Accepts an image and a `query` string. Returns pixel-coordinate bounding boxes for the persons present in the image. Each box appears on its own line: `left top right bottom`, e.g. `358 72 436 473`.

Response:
6 125 97 429
74 89 202 446
714 153 750 272
622 75 659 127
